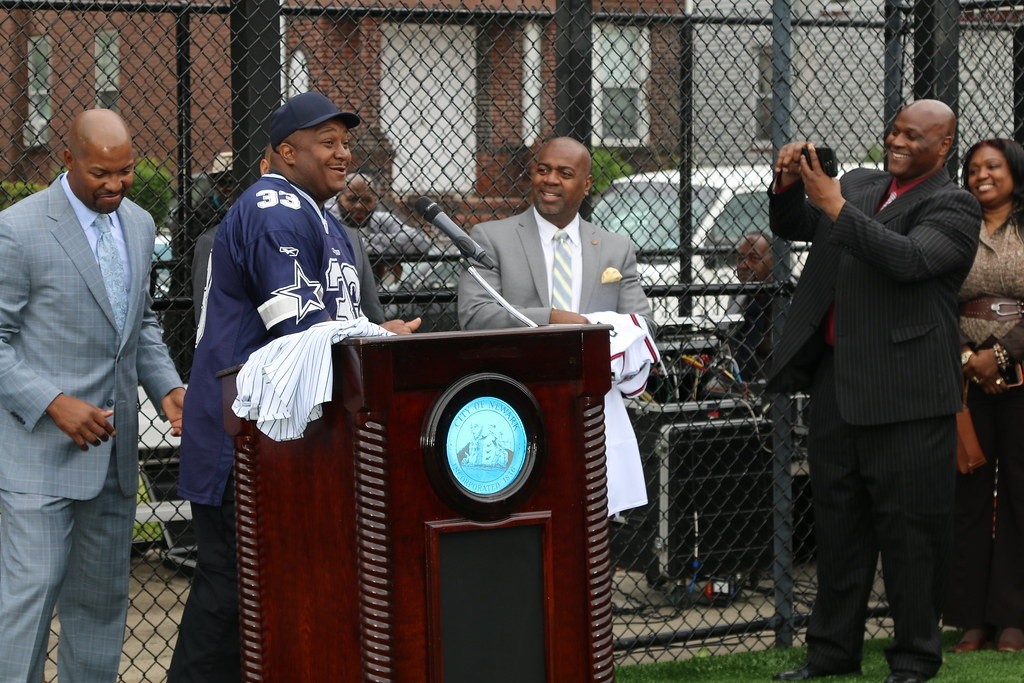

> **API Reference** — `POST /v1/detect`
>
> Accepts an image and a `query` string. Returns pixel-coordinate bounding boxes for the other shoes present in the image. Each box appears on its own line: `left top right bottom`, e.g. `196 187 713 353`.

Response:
999 623 1024 652
946 631 994 651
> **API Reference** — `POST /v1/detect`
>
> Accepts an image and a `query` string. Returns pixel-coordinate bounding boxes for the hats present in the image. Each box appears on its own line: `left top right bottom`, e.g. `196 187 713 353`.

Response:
270 92 360 150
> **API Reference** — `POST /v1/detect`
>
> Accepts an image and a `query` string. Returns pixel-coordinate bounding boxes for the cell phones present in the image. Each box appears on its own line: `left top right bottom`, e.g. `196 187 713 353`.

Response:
802 147 838 177
999 361 1023 388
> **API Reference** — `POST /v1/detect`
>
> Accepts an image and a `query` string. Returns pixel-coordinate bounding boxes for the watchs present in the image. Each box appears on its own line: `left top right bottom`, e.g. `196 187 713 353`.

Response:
961 351 972 365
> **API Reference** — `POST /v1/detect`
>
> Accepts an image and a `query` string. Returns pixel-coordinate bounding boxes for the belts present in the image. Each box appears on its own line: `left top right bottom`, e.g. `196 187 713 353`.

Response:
961 296 1024 322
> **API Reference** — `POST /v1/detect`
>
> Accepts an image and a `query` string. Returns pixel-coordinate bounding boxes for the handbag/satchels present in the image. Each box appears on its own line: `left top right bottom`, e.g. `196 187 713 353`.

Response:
952 378 985 475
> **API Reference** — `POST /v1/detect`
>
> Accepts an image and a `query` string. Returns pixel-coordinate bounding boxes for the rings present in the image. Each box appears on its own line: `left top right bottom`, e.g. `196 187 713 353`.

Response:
996 378 1003 384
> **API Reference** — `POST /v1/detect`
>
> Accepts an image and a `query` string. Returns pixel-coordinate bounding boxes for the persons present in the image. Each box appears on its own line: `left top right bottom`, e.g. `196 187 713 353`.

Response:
191 148 234 243
325 172 431 317
1 108 185 683
166 92 423 683
734 99 1023 683
456 137 659 340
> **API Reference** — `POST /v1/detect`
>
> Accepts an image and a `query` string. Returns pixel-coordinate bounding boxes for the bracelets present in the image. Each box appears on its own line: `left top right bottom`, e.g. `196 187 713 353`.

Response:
994 343 1009 369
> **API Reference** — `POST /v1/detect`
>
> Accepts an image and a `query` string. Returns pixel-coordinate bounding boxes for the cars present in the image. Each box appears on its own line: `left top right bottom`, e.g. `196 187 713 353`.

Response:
580 162 896 346
392 239 466 315
121 237 194 448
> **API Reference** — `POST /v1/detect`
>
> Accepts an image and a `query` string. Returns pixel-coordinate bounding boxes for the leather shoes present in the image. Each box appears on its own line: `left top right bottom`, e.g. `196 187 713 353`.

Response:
884 670 929 683
774 663 862 683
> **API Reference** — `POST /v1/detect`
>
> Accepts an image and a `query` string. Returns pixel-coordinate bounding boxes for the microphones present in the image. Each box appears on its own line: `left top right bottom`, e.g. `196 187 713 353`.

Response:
415 196 496 271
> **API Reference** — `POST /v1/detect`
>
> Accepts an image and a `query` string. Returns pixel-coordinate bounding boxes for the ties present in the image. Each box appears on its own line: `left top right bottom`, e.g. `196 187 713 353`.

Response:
880 191 898 212
93 213 128 334
550 227 574 313
